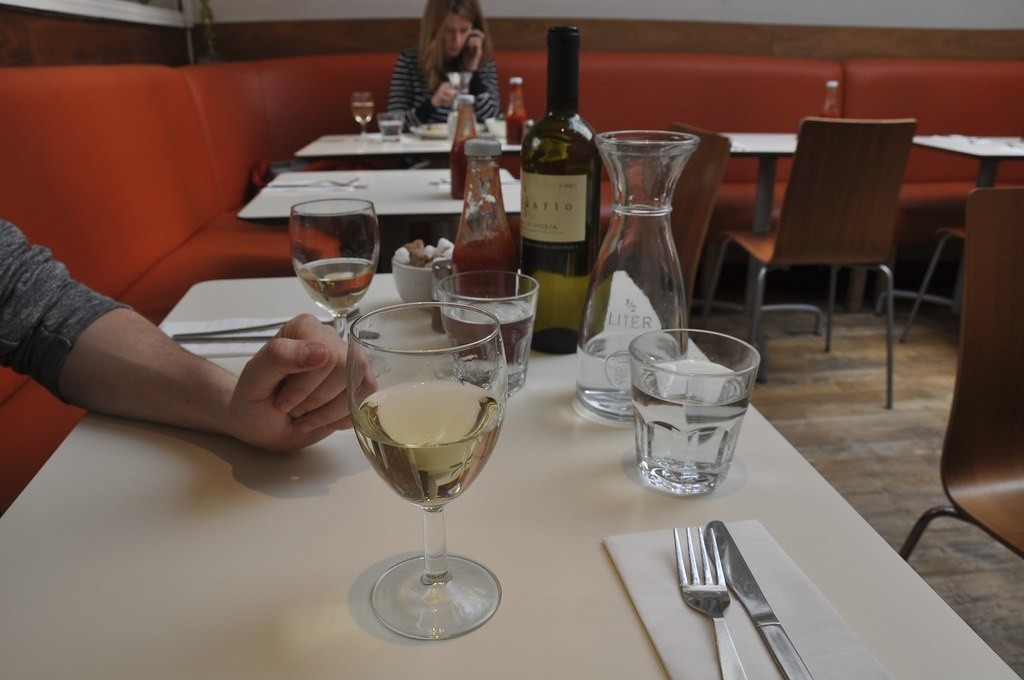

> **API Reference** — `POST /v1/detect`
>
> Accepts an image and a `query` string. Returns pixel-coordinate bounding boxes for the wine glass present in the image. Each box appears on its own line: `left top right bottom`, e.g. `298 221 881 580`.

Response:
350 91 375 137
347 301 508 640
289 198 380 349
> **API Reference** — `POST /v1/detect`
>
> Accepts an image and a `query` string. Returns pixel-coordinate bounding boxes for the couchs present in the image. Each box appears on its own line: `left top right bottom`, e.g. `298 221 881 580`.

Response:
1 53 1024 514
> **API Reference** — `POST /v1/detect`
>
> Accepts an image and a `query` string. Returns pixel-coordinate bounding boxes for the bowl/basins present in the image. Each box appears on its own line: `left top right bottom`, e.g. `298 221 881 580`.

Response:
391 260 437 305
485 117 507 140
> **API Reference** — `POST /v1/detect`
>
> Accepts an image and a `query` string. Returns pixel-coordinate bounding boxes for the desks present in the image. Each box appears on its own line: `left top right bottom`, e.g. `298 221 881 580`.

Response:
291 134 526 156
715 131 798 233
907 135 1023 211
237 170 530 215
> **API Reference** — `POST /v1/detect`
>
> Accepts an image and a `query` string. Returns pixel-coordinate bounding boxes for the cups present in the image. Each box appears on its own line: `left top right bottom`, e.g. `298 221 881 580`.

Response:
628 328 761 497
437 270 539 397
446 71 472 94
377 112 403 142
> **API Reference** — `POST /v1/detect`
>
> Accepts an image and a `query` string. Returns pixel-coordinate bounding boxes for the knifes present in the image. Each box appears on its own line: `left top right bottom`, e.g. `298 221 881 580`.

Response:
173 331 379 342
703 519 814 680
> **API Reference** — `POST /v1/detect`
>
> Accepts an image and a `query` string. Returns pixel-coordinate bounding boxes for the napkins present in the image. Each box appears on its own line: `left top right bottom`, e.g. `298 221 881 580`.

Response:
602 519 894 680
160 317 339 359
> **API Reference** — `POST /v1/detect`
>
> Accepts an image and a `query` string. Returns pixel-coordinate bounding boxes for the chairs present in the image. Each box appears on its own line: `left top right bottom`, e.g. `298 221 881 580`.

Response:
898 186 1024 560
708 116 920 407
897 225 974 343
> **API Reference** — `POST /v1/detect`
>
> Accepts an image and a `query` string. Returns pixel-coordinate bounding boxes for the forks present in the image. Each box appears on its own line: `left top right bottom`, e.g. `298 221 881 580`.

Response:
275 176 360 188
673 527 747 680
173 308 361 335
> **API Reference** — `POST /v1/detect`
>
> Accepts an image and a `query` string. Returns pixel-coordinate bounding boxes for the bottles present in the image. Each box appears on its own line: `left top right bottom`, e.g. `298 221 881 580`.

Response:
517 24 600 355
450 140 517 361
507 76 526 145
573 132 700 422
450 94 480 198
820 81 840 118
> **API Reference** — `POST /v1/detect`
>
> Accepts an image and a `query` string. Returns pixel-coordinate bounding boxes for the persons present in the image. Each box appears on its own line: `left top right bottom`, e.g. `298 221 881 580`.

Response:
0 217 379 453
386 0 501 247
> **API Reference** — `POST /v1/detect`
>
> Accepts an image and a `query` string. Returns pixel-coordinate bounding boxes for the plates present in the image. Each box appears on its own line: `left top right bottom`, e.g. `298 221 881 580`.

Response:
410 124 448 139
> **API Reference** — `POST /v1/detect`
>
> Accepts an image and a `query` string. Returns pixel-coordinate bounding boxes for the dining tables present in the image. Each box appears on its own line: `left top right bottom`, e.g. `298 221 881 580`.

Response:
1 273 1020 680
